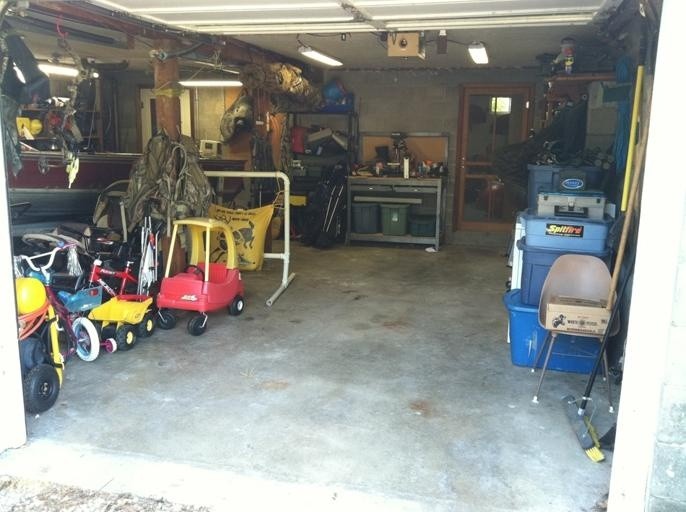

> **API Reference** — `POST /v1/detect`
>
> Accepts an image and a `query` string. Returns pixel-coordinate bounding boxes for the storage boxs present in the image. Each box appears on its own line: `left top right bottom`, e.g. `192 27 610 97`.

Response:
501 163 606 378
350 202 443 238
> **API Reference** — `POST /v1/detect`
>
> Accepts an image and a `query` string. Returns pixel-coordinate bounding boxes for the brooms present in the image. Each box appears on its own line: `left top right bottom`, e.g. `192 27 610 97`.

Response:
561 242 637 464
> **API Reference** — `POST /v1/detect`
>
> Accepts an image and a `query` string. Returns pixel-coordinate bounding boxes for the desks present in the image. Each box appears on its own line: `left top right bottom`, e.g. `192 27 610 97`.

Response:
344 165 446 249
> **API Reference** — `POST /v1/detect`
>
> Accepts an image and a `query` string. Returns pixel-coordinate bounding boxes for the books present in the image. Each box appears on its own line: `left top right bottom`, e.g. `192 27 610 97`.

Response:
307 129 336 153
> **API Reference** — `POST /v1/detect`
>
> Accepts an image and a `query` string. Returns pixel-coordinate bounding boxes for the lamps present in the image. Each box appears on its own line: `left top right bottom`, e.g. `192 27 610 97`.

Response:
447 37 490 66
295 32 344 69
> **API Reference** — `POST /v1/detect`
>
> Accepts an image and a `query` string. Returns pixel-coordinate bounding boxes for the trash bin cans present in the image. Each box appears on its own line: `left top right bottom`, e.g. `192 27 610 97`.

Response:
352 202 436 236
503 164 615 375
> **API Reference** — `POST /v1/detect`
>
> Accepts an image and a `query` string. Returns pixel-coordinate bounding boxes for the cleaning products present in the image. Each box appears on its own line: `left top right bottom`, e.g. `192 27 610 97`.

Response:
564 45 576 71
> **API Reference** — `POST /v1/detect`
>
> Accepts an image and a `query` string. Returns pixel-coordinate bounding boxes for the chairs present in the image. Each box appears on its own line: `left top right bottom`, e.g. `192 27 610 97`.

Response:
531 254 615 414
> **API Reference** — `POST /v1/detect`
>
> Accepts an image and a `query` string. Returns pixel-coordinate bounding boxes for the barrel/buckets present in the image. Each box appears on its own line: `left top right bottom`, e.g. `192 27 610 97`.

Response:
322 75 347 106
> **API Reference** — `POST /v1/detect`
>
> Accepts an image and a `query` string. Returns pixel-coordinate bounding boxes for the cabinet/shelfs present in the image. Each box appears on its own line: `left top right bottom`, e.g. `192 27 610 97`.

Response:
17 73 105 153
278 110 358 196
